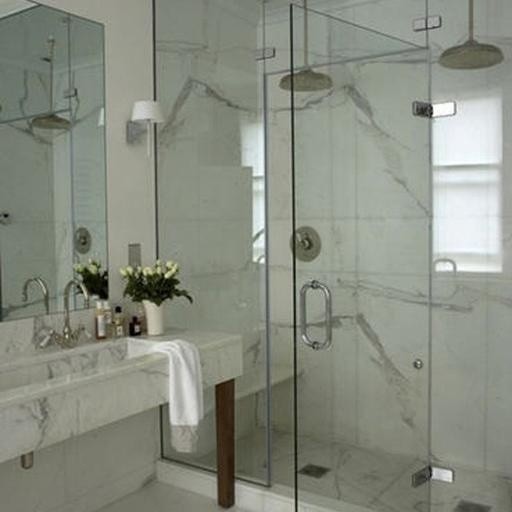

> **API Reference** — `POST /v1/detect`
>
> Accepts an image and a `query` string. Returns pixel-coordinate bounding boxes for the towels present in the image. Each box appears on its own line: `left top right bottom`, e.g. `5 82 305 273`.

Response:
151 340 207 456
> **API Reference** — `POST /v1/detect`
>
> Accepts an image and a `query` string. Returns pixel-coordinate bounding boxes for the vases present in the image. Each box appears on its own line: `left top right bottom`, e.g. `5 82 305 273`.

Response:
141 299 165 337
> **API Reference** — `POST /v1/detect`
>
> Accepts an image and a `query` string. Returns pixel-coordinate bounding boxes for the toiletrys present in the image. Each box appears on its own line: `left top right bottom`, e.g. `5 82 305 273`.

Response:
95 300 141 339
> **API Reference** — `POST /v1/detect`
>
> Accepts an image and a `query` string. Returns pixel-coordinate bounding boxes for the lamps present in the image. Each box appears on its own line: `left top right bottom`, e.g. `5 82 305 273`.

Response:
125 98 162 160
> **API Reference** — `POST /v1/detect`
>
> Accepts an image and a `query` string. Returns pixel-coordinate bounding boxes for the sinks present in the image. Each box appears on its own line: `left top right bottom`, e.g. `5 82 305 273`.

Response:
0 337 168 408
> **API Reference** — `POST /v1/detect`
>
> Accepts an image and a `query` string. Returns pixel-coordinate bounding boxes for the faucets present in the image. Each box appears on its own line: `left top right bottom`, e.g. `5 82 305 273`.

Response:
62 280 89 339
22 277 50 315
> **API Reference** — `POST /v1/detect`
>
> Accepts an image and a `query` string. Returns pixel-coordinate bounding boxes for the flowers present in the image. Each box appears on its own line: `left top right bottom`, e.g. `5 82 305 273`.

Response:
120 261 195 308
70 260 109 304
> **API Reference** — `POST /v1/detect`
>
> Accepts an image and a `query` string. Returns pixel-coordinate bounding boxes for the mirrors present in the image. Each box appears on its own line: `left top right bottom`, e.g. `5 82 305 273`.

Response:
0 0 110 323
